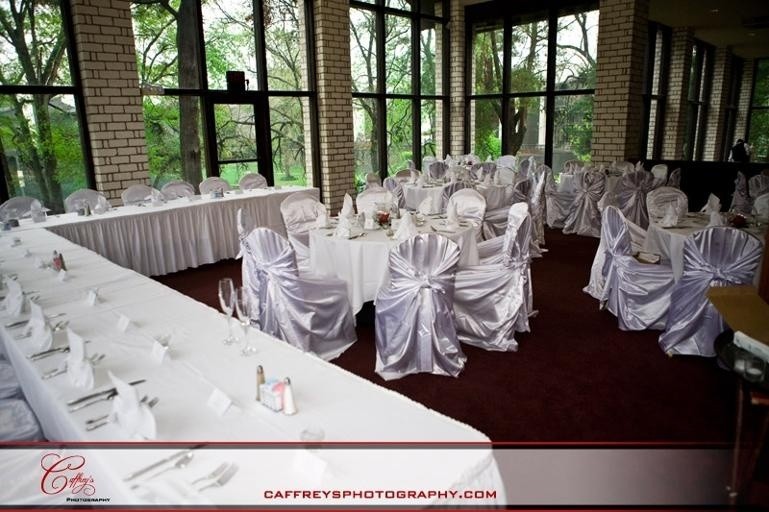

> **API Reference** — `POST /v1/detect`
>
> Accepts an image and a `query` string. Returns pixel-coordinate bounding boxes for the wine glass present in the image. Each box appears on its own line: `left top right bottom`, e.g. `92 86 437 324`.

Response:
218 278 238 347
234 288 256 356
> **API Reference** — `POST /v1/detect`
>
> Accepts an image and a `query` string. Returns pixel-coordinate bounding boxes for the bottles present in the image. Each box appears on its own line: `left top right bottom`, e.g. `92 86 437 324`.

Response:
284 376 299 416
727 204 758 227
73 200 91 217
253 363 265 401
51 247 65 271
208 187 224 199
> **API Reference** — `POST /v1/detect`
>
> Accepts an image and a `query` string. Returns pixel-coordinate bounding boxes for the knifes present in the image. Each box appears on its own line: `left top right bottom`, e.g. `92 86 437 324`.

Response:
663 225 692 229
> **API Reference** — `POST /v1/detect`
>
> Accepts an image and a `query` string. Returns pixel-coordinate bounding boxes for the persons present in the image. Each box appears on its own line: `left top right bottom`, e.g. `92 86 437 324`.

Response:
727 138 752 163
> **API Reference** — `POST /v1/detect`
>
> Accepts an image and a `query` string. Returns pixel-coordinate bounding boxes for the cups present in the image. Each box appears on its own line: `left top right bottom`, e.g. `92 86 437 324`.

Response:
1 222 10 231
8 219 19 226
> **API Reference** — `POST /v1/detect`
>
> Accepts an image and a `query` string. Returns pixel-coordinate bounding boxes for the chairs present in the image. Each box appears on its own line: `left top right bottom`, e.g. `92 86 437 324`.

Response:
1 149 769 508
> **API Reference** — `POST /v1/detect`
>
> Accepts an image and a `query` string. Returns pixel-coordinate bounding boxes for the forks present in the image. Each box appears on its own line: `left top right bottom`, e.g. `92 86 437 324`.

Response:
321 211 469 241
0 288 238 503
